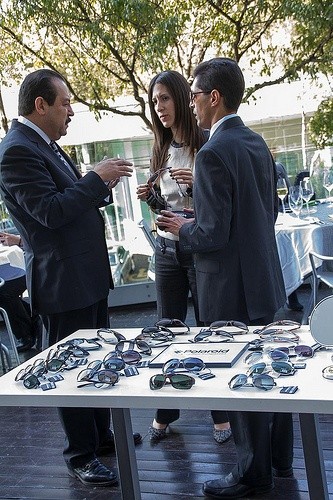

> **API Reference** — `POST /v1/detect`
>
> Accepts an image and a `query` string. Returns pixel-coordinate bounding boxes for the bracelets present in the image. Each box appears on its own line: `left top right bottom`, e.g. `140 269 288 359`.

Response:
18 236 23 247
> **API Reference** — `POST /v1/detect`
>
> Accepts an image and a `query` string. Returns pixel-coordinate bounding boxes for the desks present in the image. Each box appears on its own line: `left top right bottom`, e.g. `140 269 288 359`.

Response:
273 200 333 325
0 324 333 500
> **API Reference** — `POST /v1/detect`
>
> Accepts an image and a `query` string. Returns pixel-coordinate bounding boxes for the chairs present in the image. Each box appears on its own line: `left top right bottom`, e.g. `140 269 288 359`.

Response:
138 217 158 252
309 225 333 308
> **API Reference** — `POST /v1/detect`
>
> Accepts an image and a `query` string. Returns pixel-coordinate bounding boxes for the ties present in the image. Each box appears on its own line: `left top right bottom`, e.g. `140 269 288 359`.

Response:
49 141 67 165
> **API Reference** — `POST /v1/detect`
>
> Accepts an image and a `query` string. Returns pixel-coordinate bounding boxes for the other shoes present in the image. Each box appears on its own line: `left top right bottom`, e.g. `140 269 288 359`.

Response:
148 418 168 441
14 335 36 352
212 425 233 444
287 300 304 310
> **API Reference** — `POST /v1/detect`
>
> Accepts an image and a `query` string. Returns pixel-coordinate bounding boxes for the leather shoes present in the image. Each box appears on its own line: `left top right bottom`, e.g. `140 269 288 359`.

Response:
68 457 118 487
201 472 274 498
95 431 142 456
271 467 293 477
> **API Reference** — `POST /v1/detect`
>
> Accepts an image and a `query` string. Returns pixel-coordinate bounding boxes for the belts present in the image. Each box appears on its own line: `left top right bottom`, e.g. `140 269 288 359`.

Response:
156 235 179 249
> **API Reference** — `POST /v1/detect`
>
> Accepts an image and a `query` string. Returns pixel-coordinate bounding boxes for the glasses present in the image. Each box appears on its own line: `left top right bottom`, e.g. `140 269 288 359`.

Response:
228 320 313 392
189 91 224 102
15 318 248 390
147 166 186 208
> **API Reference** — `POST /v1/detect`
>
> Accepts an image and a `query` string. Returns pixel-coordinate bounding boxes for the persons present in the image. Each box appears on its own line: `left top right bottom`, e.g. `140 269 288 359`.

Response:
155 58 294 500
136 69 233 445
0 70 142 486
0 231 42 353
271 157 291 206
